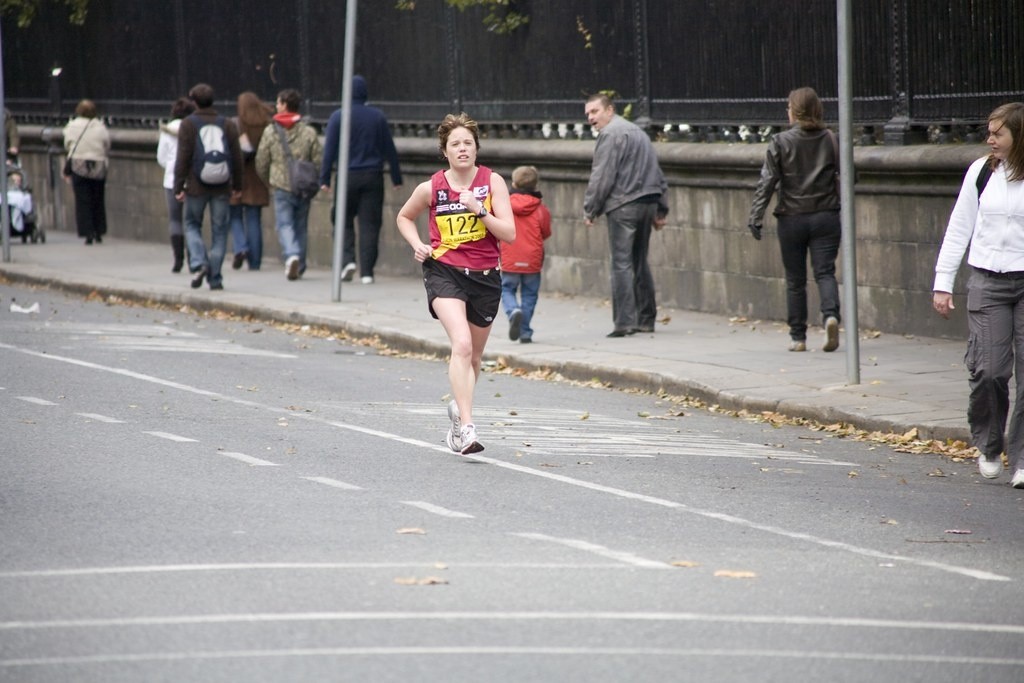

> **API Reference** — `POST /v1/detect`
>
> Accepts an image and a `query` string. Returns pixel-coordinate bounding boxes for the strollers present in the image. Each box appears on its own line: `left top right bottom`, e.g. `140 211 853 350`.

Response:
5 150 46 245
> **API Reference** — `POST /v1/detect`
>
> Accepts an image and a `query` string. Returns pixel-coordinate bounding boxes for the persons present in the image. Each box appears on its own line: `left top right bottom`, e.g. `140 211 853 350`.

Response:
497 165 552 341
320 74 404 283
933 101 1024 488
397 112 516 454
62 99 111 244
582 94 669 337
747 87 859 352
3 105 29 216
157 83 322 291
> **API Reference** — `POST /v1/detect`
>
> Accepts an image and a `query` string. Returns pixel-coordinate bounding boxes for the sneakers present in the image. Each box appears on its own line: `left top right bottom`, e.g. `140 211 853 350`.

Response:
788 340 806 351
822 316 840 352
520 338 532 343
1011 469 1024 488
340 263 356 281
509 312 523 341
446 400 462 453
285 255 299 281
361 276 374 284
979 454 1003 479
233 252 246 268
459 423 485 455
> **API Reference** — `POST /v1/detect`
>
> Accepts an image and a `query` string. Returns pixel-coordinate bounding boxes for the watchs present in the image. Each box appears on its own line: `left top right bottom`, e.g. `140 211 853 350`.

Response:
476 207 488 220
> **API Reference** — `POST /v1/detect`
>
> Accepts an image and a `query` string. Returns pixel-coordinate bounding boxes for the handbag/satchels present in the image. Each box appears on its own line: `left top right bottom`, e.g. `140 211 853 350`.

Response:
63 157 73 175
287 158 319 198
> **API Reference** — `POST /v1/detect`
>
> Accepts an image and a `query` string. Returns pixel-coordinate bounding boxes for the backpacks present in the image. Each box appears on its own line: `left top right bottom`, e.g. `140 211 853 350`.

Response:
187 113 232 187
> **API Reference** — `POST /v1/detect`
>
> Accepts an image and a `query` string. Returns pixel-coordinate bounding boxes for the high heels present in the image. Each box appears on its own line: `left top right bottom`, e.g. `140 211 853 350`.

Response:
191 265 210 287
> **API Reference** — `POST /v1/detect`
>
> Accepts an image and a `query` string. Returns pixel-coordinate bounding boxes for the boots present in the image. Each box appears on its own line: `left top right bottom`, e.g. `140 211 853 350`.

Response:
171 234 184 273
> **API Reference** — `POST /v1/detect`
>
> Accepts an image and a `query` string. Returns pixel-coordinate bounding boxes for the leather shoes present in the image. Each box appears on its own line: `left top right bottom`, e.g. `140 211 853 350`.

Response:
607 329 634 338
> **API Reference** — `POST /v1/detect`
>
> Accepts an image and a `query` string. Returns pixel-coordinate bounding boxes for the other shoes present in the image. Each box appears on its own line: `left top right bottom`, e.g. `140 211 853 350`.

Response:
94 232 101 242
85 235 93 246
